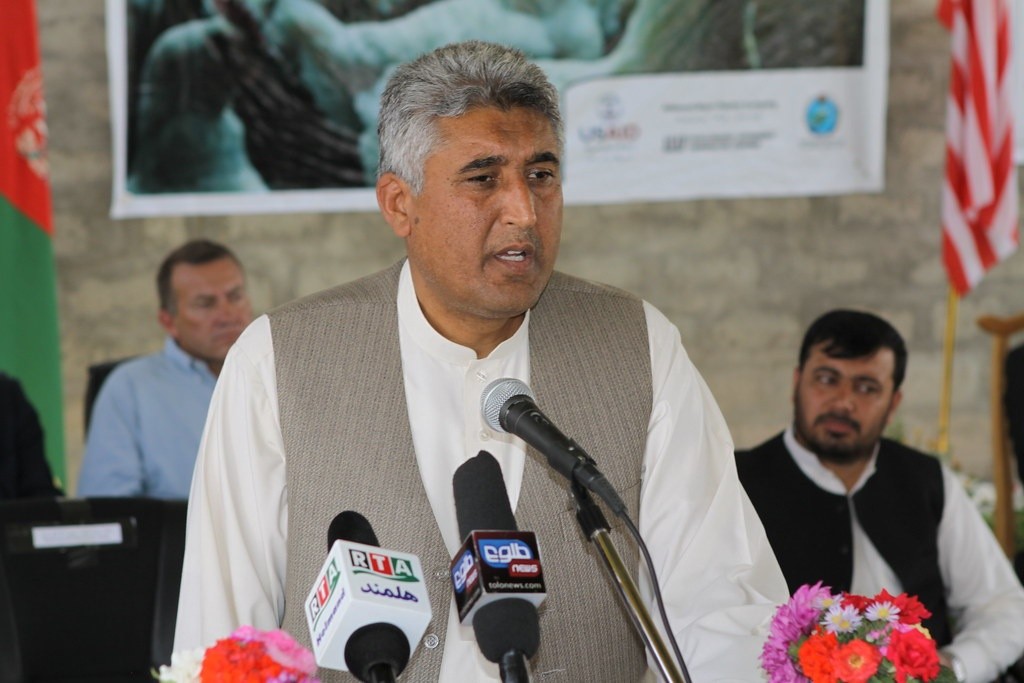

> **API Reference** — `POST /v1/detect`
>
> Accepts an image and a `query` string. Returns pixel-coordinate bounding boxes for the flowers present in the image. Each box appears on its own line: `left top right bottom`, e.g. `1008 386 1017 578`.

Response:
758 580 959 683
150 625 322 683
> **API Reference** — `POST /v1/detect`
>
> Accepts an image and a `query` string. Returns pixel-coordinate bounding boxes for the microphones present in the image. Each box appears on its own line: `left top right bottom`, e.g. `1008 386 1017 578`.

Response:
479 378 629 516
448 450 548 683
306 510 433 683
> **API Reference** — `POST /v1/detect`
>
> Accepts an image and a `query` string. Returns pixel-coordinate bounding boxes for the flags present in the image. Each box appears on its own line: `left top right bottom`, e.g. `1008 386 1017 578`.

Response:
931 0 1018 298
0 0 69 495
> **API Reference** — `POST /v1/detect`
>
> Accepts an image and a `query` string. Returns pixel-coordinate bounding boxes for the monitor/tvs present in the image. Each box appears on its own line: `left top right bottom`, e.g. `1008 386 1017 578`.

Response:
0 495 187 683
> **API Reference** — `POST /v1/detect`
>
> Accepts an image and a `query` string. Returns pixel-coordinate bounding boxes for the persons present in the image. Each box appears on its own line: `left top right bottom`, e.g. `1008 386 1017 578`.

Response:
75 236 272 500
0 373 66 519
725 308 1023 683
171 39 798 683
